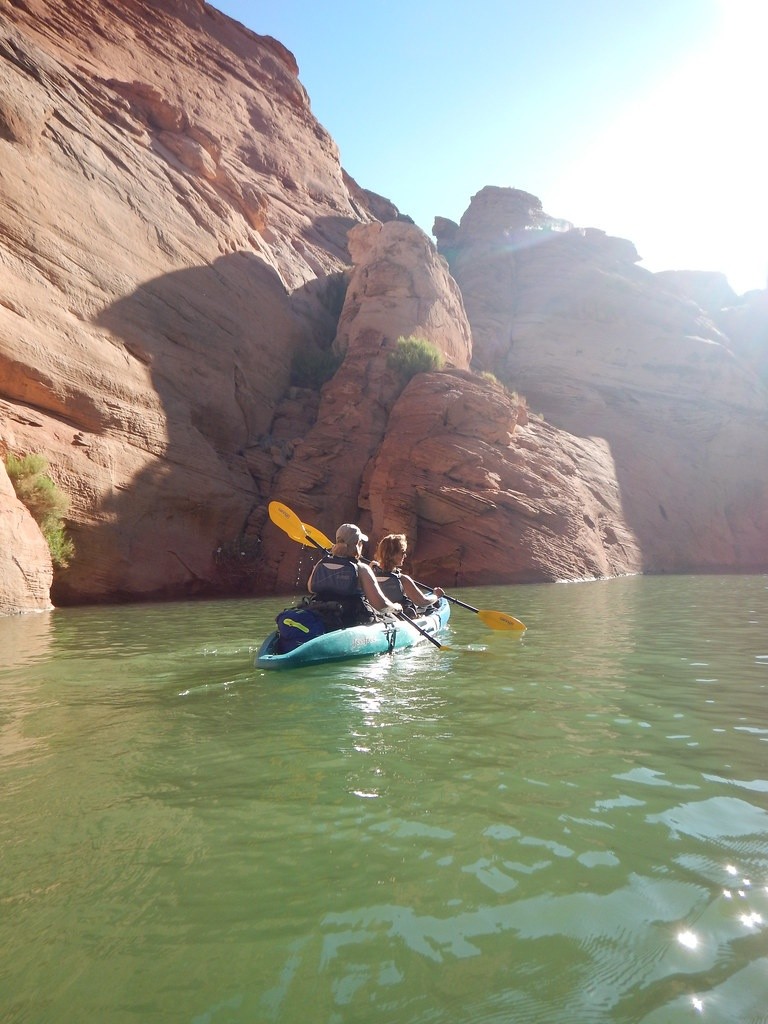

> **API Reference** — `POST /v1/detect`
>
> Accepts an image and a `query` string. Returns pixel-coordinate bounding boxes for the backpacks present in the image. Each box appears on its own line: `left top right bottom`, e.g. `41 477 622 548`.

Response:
275 609 326 658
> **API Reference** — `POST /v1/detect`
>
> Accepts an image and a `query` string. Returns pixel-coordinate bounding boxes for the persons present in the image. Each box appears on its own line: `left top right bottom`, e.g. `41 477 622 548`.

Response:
370 534 445 619
307 524 403 627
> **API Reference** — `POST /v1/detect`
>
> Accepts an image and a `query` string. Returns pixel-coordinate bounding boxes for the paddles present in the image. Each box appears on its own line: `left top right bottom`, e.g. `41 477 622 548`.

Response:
268 501 451 650
287 523 528 630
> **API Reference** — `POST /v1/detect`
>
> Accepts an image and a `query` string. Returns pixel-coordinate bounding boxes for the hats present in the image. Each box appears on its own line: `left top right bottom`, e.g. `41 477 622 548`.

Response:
335 523 369 547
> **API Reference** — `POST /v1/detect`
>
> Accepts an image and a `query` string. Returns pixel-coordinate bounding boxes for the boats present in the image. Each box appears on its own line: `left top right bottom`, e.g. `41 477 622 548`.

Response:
251 593 450 670
